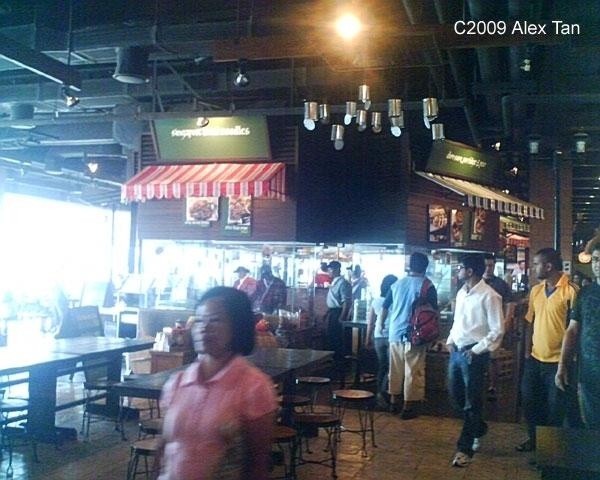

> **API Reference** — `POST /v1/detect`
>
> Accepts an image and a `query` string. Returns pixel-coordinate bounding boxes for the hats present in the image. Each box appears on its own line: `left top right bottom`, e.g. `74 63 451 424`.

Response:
233 266 250 273
346 264 361 270
325 260 341 269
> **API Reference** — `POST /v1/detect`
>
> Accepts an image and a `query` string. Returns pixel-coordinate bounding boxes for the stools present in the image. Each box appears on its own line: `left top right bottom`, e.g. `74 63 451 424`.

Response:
1 324 384 480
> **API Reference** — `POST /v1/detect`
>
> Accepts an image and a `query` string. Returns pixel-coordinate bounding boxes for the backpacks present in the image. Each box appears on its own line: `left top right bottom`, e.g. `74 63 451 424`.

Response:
405 279 442 346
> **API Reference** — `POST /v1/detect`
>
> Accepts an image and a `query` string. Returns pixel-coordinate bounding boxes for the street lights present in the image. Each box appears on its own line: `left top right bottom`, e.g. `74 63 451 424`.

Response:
526 125 589 256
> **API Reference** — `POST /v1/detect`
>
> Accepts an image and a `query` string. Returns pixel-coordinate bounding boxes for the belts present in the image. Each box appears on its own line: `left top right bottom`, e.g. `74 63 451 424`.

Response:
455 341 478 354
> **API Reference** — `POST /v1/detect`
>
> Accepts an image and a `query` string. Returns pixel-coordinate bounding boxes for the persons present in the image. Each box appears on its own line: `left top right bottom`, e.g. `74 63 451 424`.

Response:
446 240 599 465
157 287 278 479
233 265 286 317
316 252 437 417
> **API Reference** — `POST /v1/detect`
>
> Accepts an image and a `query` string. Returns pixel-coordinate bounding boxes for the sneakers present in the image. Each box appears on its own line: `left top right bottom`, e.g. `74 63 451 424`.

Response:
388 402 420 420
471 437 482 452
452 450 471 468
514 438 537 453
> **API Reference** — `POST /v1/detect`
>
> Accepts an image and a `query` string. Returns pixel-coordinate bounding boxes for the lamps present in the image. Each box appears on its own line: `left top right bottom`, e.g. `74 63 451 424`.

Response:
196 109 211 130
290 28 453 153
60 1 79 112
82 149 99 175
227 0 252 91
